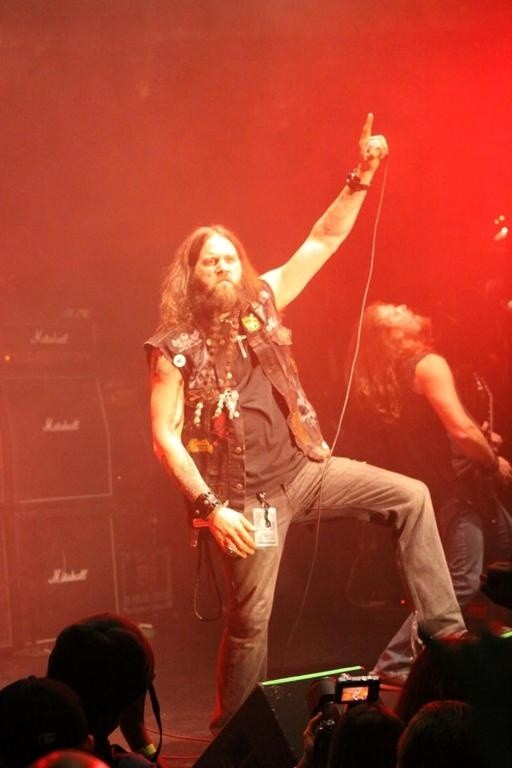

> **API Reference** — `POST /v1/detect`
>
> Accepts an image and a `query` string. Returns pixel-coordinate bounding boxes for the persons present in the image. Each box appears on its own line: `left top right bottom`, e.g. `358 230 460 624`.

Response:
292 632 512 768
140 111 481 737
346 300 512 690
1 609 161 768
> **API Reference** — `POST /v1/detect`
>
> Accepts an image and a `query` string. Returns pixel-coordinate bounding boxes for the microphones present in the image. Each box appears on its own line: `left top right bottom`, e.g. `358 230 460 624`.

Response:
367 139 381 150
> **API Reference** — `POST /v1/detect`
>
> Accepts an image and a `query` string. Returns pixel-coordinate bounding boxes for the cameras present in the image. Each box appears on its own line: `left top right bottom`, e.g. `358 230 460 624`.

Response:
308 672 380 751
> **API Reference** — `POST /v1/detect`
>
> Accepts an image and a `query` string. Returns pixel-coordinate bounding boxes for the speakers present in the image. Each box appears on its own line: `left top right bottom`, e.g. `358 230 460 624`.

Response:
93 317 176 619
0 308 115 501
194 665 367 767
2 497 120 646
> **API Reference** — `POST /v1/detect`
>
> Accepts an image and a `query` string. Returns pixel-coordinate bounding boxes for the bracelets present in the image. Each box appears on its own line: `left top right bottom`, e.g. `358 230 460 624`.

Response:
190 490 222 520
487 459 500 473
